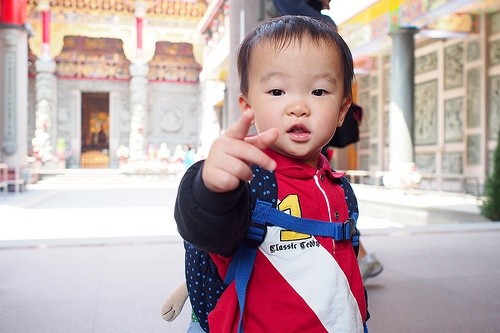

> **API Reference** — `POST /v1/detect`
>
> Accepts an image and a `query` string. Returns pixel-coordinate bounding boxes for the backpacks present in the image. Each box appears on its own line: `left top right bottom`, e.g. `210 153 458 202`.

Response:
184 162 370 333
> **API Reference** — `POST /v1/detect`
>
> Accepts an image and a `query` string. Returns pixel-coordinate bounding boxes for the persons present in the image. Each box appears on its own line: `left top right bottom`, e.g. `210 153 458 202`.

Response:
273 0 382 284
174 15 371 333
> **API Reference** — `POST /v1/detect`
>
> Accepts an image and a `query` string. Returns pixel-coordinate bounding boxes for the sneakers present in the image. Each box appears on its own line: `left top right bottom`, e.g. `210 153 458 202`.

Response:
358 254 383 283
